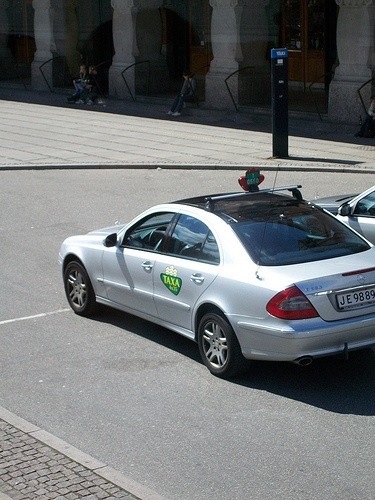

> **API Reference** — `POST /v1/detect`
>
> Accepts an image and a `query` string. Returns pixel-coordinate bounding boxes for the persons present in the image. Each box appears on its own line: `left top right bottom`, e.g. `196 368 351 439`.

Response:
165 72 197 116
66 64 103 105
354 96 375 138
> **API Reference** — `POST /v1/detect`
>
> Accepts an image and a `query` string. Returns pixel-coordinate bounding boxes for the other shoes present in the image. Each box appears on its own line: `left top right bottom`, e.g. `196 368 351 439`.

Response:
165 110 174 115
172 112 182 116
67 96 79 101
86 100 94 105
74 100 84 104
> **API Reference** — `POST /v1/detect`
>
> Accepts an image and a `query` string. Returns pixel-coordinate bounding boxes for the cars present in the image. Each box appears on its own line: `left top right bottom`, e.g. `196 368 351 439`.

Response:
57 168 375 378
307 183 375 243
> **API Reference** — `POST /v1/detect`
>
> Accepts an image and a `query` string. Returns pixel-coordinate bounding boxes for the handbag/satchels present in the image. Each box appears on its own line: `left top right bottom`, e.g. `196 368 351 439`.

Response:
73 72 93 94
